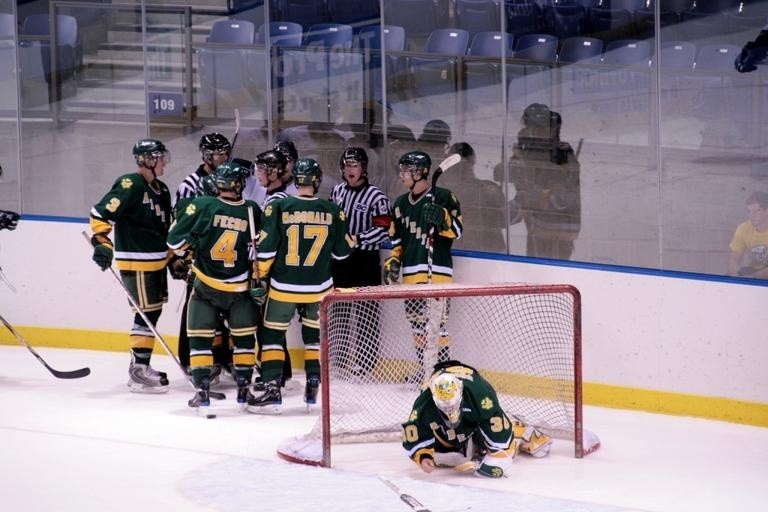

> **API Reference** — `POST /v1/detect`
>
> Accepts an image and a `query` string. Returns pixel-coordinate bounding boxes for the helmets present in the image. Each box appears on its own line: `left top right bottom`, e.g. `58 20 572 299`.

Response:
429 374 461 415
132 139 167 165
339 148 366 173
198 132 321 192
399 151 430 175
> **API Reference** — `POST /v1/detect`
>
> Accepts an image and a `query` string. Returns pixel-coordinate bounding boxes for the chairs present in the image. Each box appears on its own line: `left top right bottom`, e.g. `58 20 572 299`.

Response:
25 10 85 74
0 12 18 43
193 0 768 168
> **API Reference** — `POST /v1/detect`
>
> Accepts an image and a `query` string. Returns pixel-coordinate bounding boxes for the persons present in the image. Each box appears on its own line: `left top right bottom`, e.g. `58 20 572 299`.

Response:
170 132 233 385
728 190 768 283
0 210 21 232
166 161 261 411
381 151 463 391
326 147 391 380
733 26 768 74
245 156 356 416
399 357 554 482
251 149 296 396
274 95 584 263
84 137 172 395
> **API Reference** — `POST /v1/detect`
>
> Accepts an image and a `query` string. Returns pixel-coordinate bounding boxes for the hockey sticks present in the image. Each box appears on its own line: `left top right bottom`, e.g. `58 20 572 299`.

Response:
82 232 226 400
428 153 461 285
1 313 90 378
229 108 240 152
198 311 261 418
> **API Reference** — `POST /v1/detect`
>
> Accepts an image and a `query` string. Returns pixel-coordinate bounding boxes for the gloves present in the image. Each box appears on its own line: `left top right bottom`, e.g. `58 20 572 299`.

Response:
0 210 19 231
422 203 444 226
93 243 112 271
167 258 188 279
382 256 401 285
250 278 266 305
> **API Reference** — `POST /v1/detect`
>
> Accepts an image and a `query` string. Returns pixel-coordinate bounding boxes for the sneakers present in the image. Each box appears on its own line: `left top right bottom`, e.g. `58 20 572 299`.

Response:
188 364 291 407
129 363 168 386
302 375 320 404
